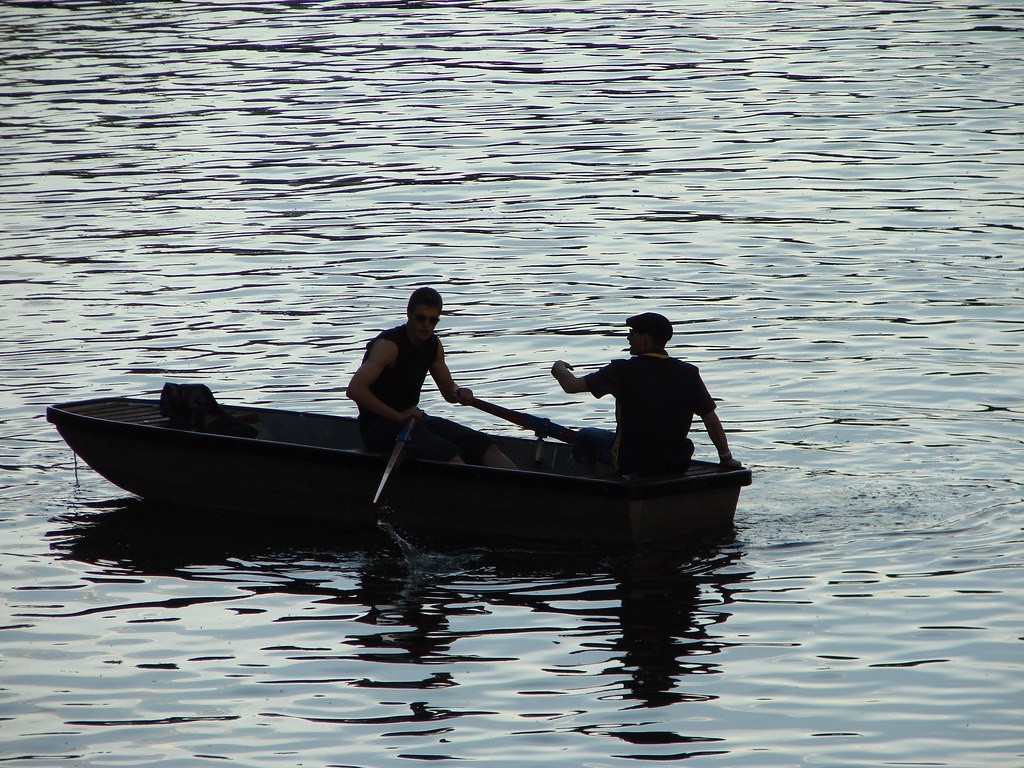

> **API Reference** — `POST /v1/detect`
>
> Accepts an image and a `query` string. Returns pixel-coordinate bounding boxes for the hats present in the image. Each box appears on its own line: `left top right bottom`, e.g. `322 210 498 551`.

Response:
626 312 673 341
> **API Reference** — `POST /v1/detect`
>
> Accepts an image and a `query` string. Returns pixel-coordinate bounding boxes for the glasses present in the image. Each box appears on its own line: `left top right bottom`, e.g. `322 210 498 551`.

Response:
417 315 441 326
629 329 649 337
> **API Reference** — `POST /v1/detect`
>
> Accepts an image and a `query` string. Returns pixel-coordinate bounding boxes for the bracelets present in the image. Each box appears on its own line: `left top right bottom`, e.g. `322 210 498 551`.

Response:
719 449 732 461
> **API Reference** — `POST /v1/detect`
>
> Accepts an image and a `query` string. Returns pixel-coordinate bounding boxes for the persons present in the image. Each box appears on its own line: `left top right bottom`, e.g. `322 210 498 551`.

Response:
551 313 743 482
346 286 521 470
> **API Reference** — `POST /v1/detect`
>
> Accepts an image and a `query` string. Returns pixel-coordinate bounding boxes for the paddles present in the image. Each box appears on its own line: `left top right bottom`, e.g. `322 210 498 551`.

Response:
369 413 420 510
460 395 695 460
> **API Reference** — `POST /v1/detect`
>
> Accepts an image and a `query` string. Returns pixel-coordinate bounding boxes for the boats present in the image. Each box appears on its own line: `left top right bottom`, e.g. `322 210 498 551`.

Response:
46 383 753 552
